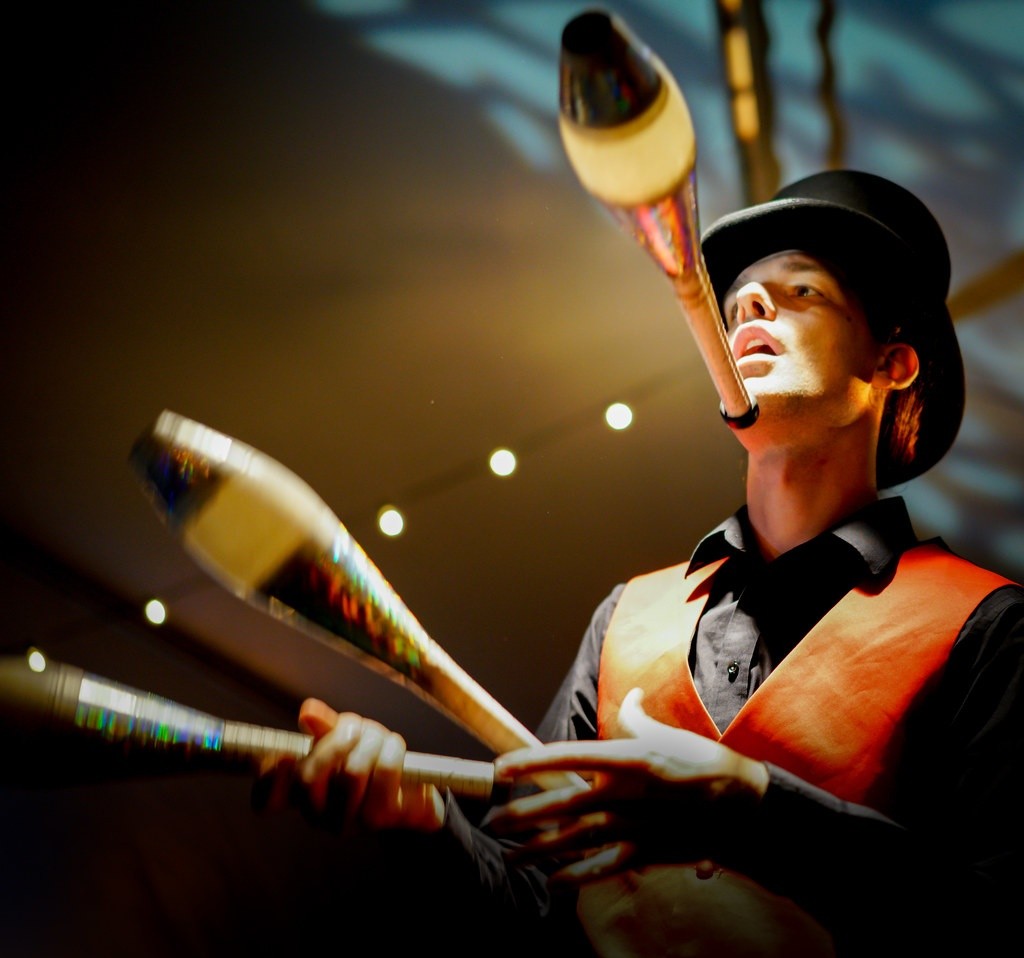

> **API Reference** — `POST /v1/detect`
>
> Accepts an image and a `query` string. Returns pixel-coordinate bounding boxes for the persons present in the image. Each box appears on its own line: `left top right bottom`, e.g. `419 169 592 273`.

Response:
262 167 1024 958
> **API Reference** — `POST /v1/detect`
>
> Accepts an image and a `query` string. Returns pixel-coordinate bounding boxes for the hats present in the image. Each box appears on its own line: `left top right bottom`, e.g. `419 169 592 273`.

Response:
703 169 966 490
